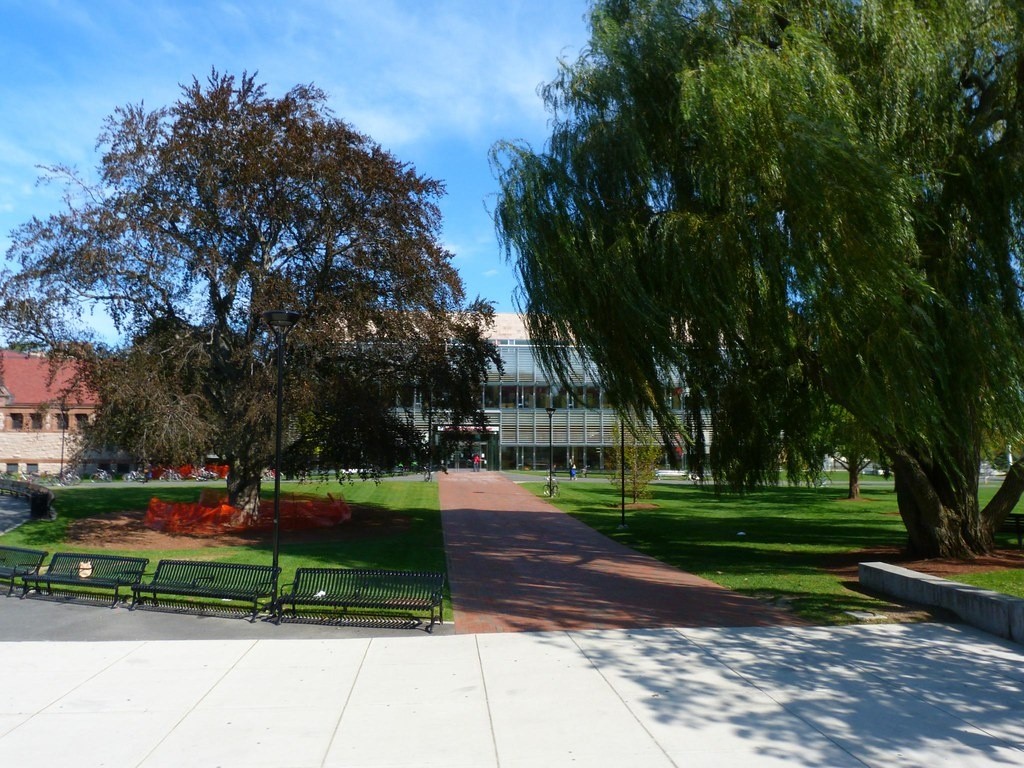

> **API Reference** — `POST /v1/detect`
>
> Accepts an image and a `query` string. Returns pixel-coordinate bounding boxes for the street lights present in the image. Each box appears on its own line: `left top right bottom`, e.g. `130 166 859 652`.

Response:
545 407 557 498
261 309 302 617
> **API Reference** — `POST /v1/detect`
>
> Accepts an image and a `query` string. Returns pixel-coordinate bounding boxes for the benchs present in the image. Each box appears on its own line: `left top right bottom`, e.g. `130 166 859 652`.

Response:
19 552 149 610
0 479 55 518
996 513 1024 546
273 567 445 634
129 560 282 623
0 545 49 598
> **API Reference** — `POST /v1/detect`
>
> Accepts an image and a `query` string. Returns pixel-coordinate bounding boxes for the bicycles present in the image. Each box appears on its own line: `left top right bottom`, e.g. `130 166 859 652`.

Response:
399 461 432 482
542 476 561 498
2 466 228 488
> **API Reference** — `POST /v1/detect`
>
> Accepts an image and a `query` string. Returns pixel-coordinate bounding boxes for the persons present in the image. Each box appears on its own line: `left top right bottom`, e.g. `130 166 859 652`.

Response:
571 463 576 477
473 453 480 471
568 458 577 480
199 465 208 477
144 461 152 482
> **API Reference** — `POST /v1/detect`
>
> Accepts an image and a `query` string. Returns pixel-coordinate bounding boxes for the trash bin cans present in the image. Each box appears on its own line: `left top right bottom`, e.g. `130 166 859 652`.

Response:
30 491 49 519
